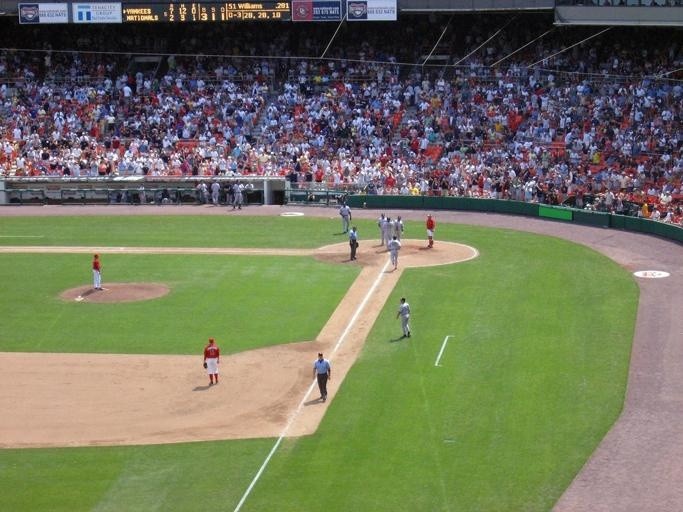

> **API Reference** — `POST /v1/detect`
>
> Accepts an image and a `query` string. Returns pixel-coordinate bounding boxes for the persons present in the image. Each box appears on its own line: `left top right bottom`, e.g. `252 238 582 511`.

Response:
340 202 351 233
203 337 220 385
348 226 359 260
378 213 404 246
92 254 103 290
0 21 683 224
426 214 435 248
313 352 331 401
387 235 402 270
395 297 411 339
